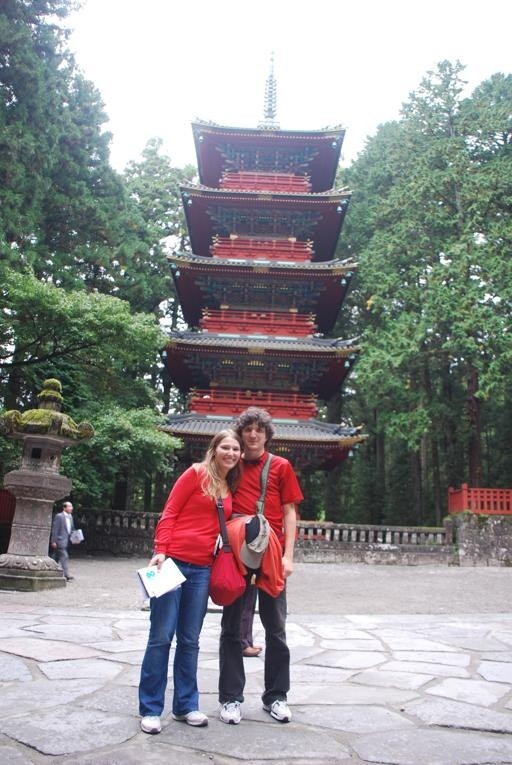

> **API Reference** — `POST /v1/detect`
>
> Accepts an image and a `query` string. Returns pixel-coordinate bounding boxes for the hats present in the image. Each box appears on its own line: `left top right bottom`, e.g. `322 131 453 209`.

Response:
239 514 271 570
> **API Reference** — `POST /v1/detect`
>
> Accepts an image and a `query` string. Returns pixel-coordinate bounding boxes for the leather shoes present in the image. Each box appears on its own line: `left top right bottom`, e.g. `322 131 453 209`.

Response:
243 647 261 656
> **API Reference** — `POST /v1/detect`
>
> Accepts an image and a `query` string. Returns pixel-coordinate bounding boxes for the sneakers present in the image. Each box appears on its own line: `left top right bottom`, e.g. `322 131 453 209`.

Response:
141 715 162 733
220 700 242 724
172 710 208 726
262 700 292 721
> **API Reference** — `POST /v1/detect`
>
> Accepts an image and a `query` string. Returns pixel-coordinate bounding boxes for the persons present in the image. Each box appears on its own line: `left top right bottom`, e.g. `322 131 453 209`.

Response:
213 406 305 726
136 428 241 736
50 500 81 581
239 580 269 658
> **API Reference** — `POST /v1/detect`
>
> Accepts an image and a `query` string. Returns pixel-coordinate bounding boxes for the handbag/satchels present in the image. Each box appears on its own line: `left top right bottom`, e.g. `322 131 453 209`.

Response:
214 515 270 586
209 546 246 605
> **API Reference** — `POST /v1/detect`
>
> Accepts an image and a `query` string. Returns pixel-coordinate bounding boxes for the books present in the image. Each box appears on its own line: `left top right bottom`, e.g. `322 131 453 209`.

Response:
70 529 85 545
137 557 187 599
